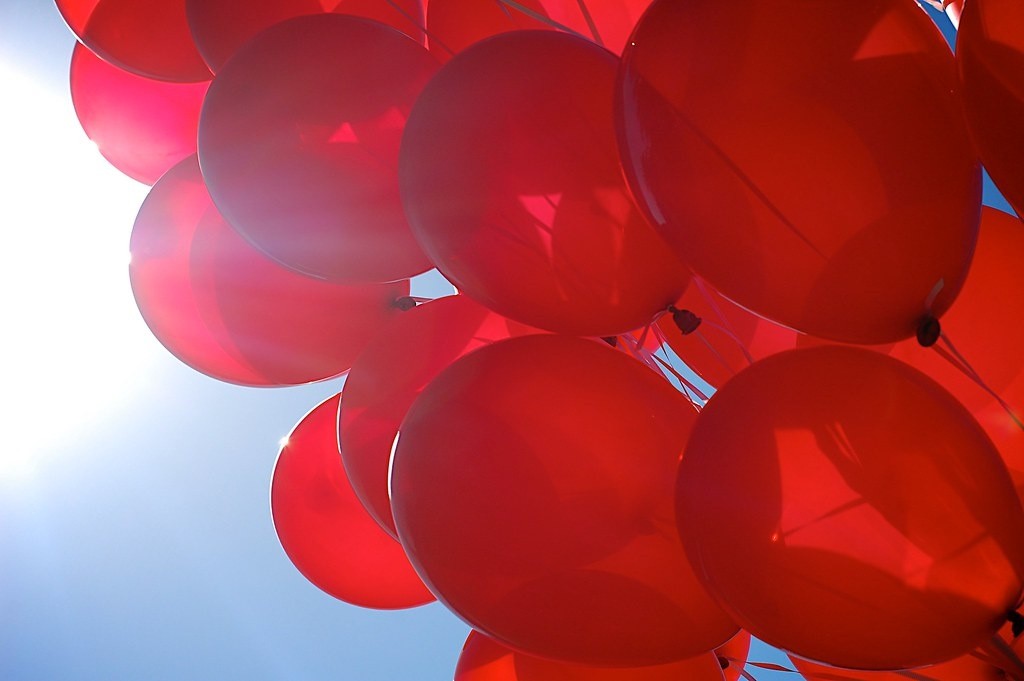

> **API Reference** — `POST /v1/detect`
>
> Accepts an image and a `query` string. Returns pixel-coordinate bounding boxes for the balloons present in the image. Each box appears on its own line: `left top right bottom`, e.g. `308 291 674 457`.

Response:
52 0 1024 681
673 348 1024 671
398 28 702 334
128 153 408 387
